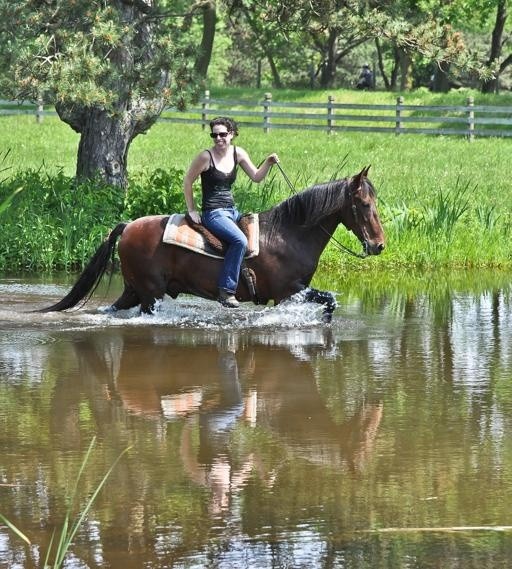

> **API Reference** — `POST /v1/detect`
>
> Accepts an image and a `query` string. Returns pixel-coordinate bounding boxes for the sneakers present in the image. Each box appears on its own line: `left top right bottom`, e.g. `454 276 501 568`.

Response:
218 287 240 308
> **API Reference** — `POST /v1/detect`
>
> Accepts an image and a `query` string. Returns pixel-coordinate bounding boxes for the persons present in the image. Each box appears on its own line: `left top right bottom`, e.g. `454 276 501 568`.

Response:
184 116 279 308
180 347 283 520
359 63 373 87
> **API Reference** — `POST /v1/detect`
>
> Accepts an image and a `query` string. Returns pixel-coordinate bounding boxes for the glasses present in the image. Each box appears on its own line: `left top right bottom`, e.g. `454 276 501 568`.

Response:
209 131 231 139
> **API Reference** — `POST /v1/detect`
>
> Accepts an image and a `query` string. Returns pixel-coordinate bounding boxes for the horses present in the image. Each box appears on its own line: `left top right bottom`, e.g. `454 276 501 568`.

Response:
20 164 386 324
68 323 386 480
